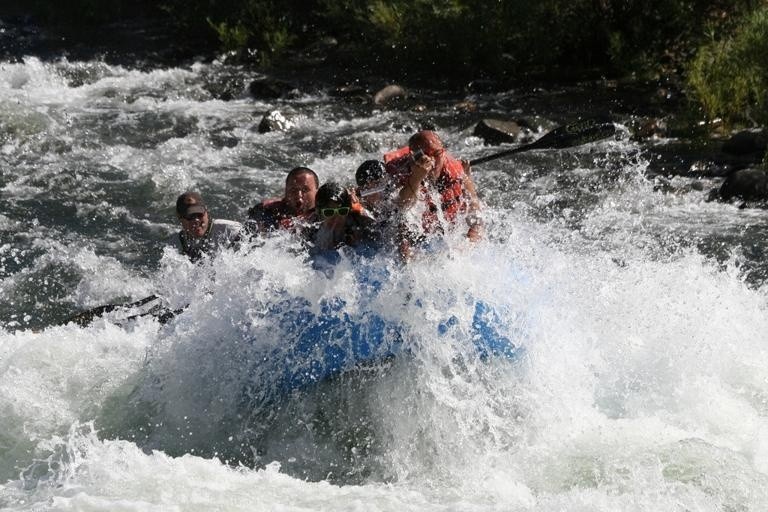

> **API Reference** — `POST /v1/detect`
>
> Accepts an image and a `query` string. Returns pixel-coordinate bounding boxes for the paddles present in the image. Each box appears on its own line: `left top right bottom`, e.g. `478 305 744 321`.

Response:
470 113 616 165
58 294 157 328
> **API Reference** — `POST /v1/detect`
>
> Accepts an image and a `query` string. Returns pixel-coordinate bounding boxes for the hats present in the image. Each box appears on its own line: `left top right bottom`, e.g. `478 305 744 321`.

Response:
176 191 207 218
355 159 388 197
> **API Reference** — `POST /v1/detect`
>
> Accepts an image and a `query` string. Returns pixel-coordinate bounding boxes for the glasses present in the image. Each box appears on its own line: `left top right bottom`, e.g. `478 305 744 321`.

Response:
318 207 351 219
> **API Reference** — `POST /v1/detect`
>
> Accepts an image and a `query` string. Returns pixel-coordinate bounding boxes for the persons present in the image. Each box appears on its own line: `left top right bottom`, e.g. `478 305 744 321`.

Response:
246 129 483 260
146 192 246 272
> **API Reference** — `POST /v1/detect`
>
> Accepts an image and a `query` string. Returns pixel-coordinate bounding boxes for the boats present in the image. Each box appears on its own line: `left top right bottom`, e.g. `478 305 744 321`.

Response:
198 253 522 421
